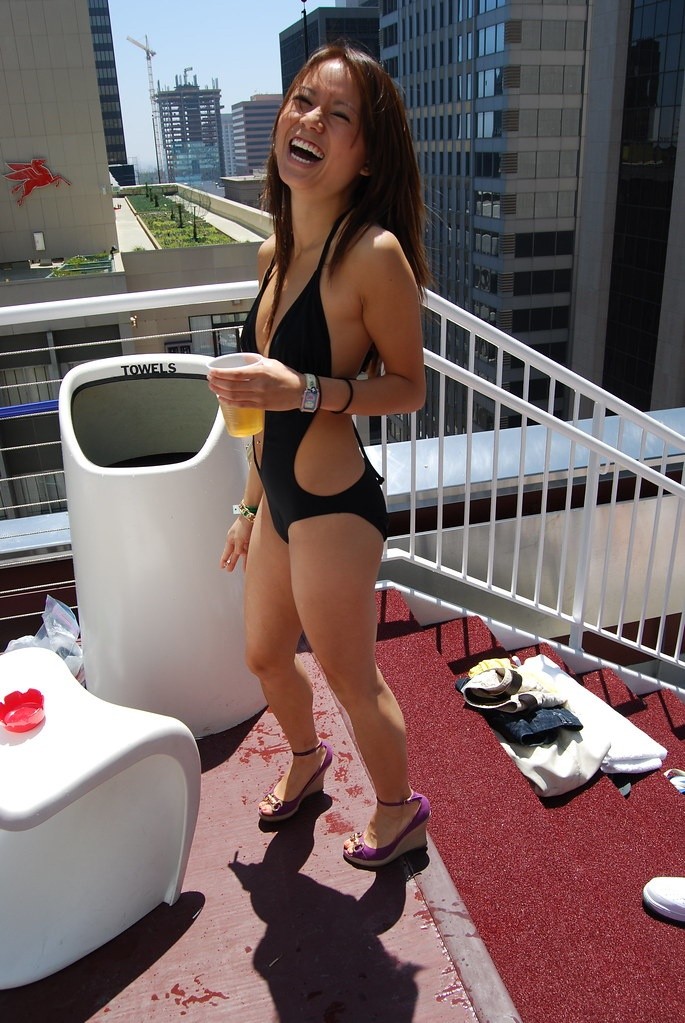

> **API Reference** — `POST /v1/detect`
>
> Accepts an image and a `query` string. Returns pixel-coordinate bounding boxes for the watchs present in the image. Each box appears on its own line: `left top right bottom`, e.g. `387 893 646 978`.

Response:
300 374 318 412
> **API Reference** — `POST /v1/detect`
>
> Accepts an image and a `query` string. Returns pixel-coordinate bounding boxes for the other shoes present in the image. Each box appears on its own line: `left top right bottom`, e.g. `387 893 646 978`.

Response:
642 876 685 922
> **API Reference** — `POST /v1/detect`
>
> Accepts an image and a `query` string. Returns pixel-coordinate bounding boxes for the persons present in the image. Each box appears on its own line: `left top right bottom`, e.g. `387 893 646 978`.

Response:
206 35 431 865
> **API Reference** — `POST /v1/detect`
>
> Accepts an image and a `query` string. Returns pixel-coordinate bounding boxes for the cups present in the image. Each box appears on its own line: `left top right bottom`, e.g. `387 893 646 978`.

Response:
206 352 265 438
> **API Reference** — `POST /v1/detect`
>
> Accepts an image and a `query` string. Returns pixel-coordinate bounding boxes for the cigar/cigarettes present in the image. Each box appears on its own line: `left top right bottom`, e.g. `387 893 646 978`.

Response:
223 558 230 568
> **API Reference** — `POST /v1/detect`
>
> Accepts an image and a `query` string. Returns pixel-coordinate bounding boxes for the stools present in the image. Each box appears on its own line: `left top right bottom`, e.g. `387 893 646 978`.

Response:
0 645 202 991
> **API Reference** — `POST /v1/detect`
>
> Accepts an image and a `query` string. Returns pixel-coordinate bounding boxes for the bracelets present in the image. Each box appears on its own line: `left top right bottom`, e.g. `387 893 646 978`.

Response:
233 499 258 523
332 377 354 414
313 373 323 413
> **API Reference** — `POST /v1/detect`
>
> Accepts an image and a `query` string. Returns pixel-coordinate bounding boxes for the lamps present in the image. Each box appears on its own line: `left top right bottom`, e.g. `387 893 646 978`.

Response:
110 245 117 260
234 301 241 304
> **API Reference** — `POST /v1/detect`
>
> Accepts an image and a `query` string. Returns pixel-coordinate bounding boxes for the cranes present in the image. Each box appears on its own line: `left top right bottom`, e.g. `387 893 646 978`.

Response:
126 33 164 184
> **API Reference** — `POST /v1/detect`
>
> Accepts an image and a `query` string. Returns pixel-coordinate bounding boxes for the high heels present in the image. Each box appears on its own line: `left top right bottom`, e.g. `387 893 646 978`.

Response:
258 740 333 821
342 788 432 866
